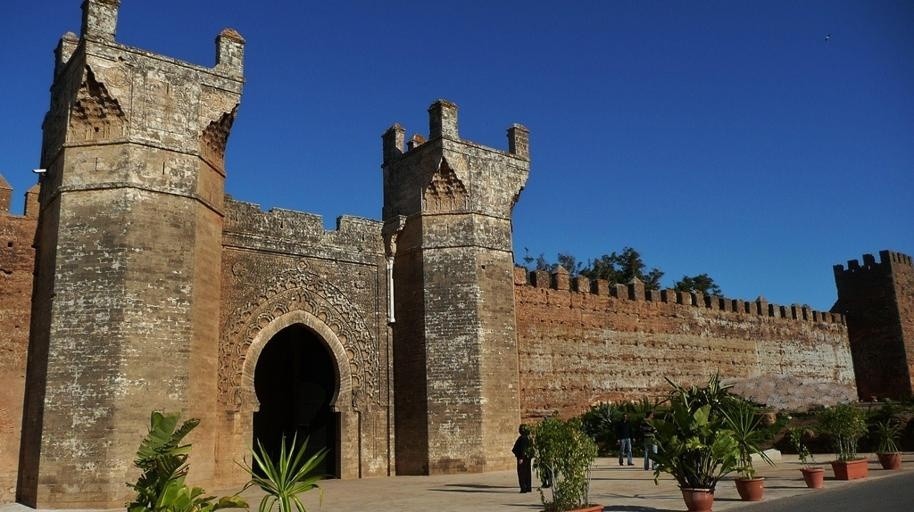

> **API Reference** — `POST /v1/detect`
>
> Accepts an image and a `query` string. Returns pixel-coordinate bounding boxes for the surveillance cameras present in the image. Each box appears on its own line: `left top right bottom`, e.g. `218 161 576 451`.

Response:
32 168 47 173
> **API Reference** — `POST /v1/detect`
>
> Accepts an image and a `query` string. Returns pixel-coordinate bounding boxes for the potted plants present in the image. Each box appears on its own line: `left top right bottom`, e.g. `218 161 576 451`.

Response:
639 368 755 512
716 399 777 500
778 410 831 488
523 414 604 512
863 398 914 470
816 401 871 480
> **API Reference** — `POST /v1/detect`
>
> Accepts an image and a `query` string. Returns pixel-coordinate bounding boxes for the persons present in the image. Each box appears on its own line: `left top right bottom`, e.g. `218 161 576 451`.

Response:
539 472 552 488
615 412 636 465
639 410 661 470
511 423 536 493
